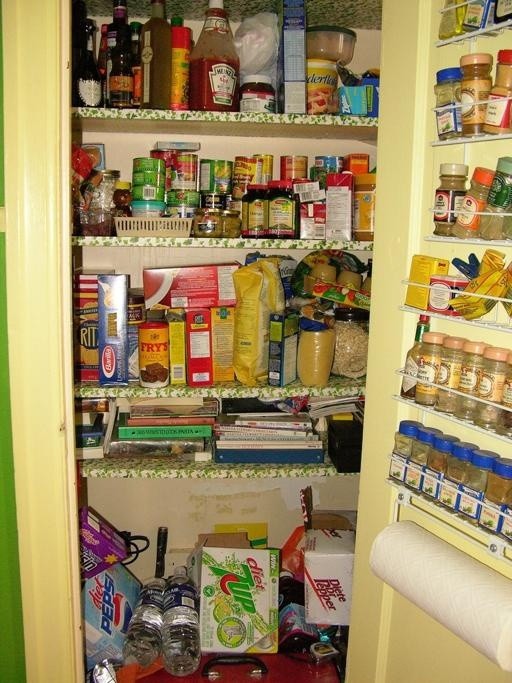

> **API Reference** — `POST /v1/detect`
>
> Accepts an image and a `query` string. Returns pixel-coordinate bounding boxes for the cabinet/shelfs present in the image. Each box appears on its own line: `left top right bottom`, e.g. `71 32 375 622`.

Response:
388 0 512 564
68 102 379 480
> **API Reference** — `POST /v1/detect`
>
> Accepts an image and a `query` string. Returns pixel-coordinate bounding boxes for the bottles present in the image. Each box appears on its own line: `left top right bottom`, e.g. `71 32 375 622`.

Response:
400 312 511 430
194 0 240 110
434 51 512 140
123 578 166 668
164 564 203 677
278 570 318 655
434 154 511 241
73 0 191 109
389 420 512 542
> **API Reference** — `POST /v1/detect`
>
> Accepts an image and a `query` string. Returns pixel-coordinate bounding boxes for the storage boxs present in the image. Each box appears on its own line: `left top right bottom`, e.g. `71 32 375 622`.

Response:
81 562 142 676
77 504 129 589
296 485 357 628
197 530 282 655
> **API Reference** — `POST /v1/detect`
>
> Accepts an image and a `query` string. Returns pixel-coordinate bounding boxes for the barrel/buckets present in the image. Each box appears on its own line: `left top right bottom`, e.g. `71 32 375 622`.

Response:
303 58 340 117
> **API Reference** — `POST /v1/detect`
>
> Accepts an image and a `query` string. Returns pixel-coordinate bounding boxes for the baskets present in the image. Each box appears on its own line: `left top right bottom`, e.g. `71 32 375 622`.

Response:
112 217 193 238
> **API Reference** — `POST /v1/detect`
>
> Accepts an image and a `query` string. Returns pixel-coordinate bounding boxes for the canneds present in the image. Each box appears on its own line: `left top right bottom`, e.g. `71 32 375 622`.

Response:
240 75 275 115
434 50 512 141
388 420 512 541
414 332 512 428
78 151 376 242
432 156 512 241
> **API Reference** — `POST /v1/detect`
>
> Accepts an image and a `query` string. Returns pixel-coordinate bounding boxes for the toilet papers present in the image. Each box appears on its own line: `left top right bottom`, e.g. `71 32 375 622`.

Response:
368 519 512 673
